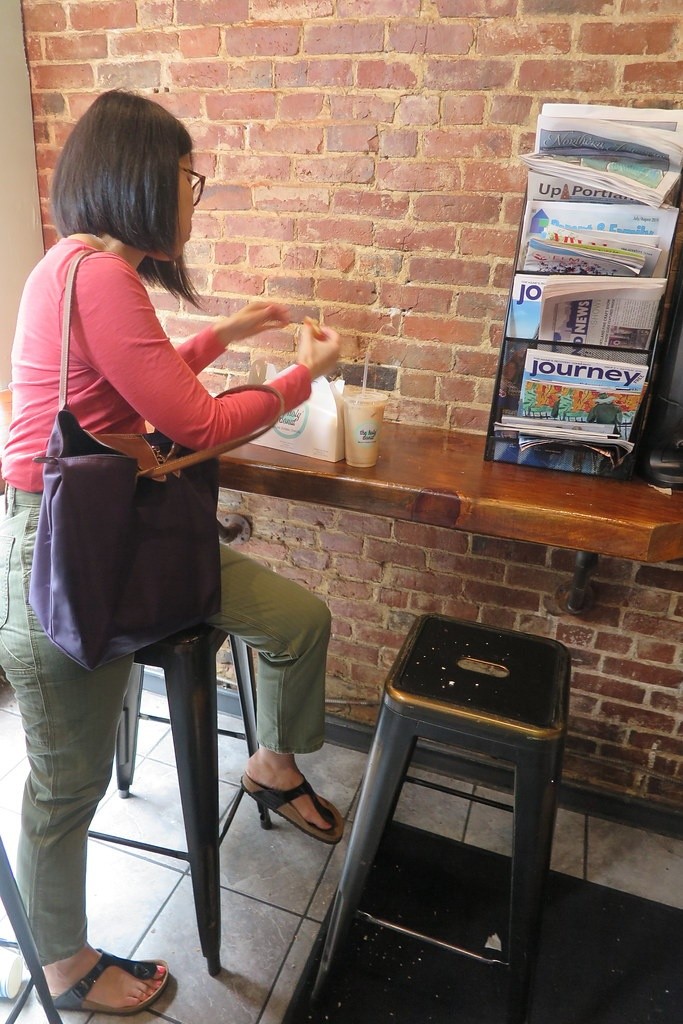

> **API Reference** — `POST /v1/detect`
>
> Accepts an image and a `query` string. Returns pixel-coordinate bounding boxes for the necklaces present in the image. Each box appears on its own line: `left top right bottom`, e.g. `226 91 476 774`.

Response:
91 233 110 250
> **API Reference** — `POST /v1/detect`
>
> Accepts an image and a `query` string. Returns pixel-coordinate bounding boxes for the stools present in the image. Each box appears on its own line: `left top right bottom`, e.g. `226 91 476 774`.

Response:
86 624 272 977
324 612 574 1024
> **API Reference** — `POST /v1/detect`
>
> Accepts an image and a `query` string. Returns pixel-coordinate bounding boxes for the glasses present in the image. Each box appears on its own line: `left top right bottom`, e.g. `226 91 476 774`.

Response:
179 164 205 206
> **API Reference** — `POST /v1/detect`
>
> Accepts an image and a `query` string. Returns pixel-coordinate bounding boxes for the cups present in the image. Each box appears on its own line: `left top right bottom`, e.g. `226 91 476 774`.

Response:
341 390 390 468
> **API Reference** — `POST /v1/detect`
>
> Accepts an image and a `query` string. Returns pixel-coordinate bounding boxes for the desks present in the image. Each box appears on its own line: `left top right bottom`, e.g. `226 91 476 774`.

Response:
0 392 683 617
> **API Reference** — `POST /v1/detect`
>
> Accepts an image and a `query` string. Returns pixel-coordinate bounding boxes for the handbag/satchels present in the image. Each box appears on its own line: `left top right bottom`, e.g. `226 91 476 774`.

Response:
29 250 285 670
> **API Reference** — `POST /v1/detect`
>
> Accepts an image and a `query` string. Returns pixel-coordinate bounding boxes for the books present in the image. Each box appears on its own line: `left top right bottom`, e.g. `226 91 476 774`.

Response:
491 225 662 477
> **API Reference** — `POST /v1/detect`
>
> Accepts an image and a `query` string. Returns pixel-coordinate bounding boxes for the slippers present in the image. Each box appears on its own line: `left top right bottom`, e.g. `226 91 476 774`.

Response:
240 771 344 844
35 948 168 1013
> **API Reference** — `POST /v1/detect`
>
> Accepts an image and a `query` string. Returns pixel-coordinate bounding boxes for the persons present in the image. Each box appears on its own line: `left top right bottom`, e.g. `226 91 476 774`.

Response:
0 91 346 1016
